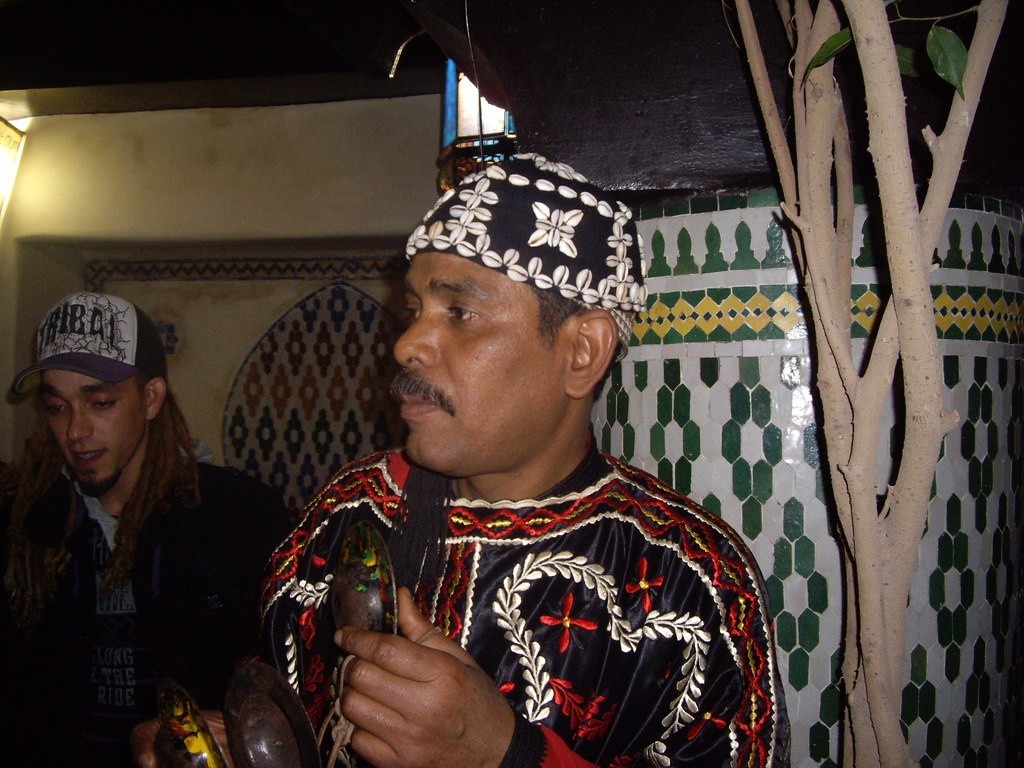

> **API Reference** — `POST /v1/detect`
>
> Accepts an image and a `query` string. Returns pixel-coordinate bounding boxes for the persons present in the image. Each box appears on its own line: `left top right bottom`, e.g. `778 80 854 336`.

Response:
3 292 293 767
117 148 796 768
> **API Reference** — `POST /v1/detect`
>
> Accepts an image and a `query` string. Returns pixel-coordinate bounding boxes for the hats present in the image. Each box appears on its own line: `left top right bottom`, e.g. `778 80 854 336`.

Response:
406 151 654 314
13 292 165 393
